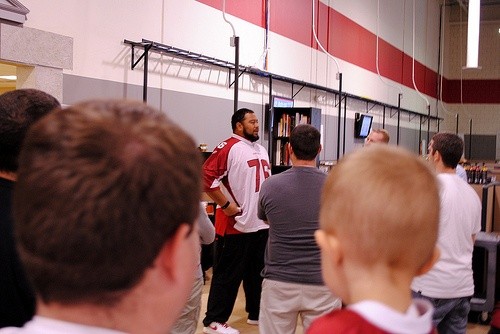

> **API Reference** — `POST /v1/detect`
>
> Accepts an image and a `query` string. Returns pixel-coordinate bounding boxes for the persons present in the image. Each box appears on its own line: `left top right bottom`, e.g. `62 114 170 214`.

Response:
0 89 63 330
202 108 273 334
170 202 215 334
0 97 202 334
367 129 390 145
303 144 442 334
409 132 482 334
258 125 343 334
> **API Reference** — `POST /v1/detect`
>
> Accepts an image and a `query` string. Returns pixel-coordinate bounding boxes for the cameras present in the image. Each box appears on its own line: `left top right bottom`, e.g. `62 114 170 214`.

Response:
358 114 373 138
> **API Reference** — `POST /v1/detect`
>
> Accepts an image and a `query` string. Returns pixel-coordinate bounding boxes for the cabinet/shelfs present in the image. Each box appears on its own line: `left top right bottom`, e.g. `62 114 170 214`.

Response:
268 105 322 175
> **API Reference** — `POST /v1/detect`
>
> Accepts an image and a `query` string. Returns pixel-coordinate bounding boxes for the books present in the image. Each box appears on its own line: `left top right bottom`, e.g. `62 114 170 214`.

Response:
273 113 311 167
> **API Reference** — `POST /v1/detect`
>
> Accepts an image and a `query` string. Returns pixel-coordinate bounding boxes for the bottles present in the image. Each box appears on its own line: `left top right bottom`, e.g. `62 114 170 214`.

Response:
464 162 488 184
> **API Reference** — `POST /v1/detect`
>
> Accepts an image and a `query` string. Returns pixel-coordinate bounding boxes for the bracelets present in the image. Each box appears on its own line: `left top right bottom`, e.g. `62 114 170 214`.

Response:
221 201 230 210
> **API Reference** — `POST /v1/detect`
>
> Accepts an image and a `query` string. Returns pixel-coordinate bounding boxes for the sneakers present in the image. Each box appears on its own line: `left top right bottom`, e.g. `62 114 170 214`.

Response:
203 321 240 334
247 319 259 325
